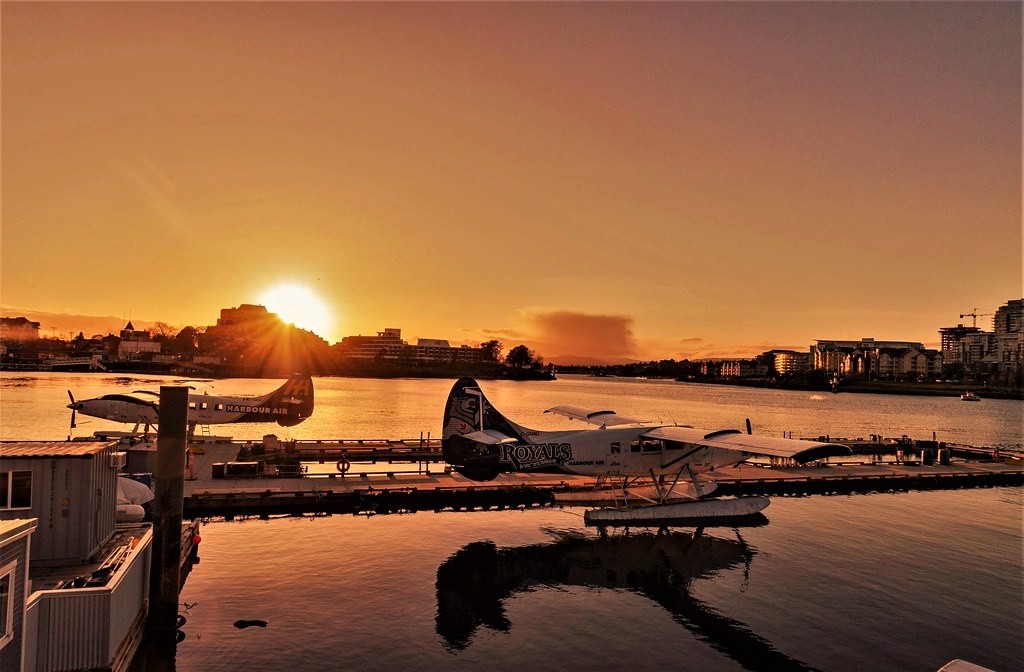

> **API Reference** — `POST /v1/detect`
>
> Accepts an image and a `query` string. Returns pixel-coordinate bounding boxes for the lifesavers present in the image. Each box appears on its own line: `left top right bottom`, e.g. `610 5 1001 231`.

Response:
336 459 351 472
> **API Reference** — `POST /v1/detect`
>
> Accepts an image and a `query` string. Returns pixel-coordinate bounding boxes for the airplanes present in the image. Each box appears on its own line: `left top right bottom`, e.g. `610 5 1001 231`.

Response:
440 373 855 504
59 372 314 438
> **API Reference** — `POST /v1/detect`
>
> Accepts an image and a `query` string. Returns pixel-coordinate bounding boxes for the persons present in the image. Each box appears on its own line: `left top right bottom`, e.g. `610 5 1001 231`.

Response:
993 447 1000 463
191 531 201 558
